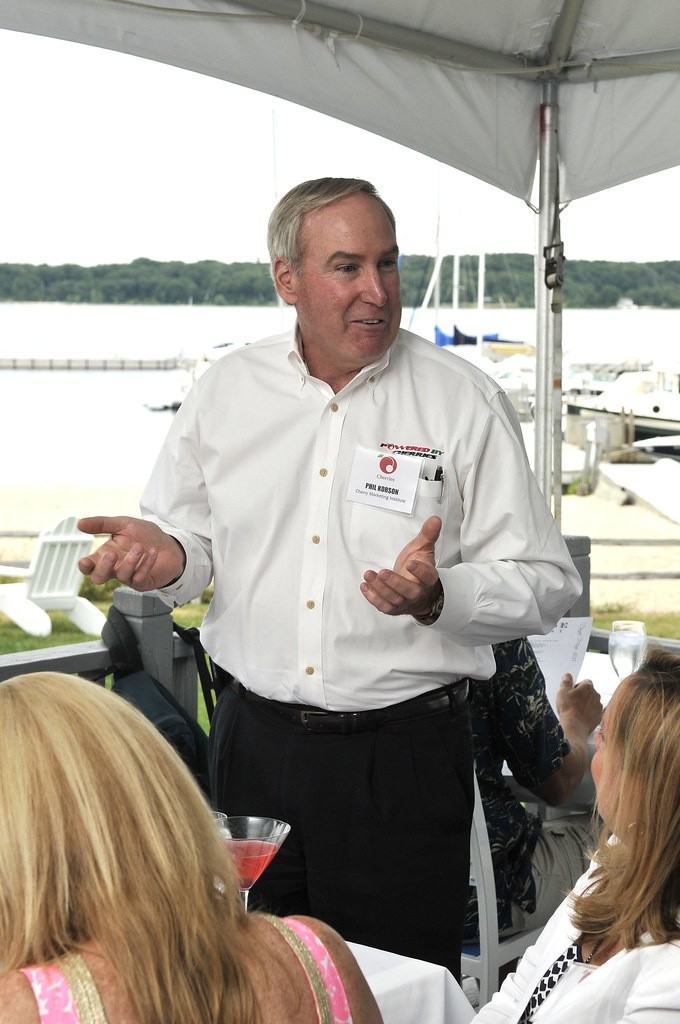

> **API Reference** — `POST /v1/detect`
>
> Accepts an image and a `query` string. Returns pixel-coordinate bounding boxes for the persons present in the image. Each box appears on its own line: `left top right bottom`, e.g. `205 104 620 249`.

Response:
78 175 584 991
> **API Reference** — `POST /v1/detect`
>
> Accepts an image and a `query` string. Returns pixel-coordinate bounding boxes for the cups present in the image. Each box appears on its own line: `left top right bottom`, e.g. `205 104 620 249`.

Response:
211 812 232 892
608 621 646 681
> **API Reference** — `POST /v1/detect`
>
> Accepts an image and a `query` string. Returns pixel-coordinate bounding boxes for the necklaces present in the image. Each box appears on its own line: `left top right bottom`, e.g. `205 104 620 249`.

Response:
586 937 604 964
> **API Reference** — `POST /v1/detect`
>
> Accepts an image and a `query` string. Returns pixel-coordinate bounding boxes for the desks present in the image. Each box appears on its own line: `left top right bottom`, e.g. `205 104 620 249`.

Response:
501 651 622 893
344 939 477 1024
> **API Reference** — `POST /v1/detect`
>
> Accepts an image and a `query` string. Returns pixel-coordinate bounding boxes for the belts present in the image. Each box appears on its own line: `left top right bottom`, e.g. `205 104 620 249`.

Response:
232 683 473 733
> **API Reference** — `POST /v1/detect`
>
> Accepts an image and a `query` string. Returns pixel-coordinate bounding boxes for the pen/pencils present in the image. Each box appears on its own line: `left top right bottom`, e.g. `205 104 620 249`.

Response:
434 465 444 480
425 476 429 481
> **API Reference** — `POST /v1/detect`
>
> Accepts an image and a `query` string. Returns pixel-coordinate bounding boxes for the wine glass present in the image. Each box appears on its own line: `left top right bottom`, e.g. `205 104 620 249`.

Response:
215 817 291 915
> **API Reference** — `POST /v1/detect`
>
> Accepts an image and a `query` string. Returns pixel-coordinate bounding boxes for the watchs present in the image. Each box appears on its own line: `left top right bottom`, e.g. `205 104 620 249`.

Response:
0 635 680 1024
415 590 444 622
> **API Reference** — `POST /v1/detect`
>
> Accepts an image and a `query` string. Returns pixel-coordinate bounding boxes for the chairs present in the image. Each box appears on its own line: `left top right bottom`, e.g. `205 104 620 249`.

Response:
460 770 547 1012
0 514 107 637
